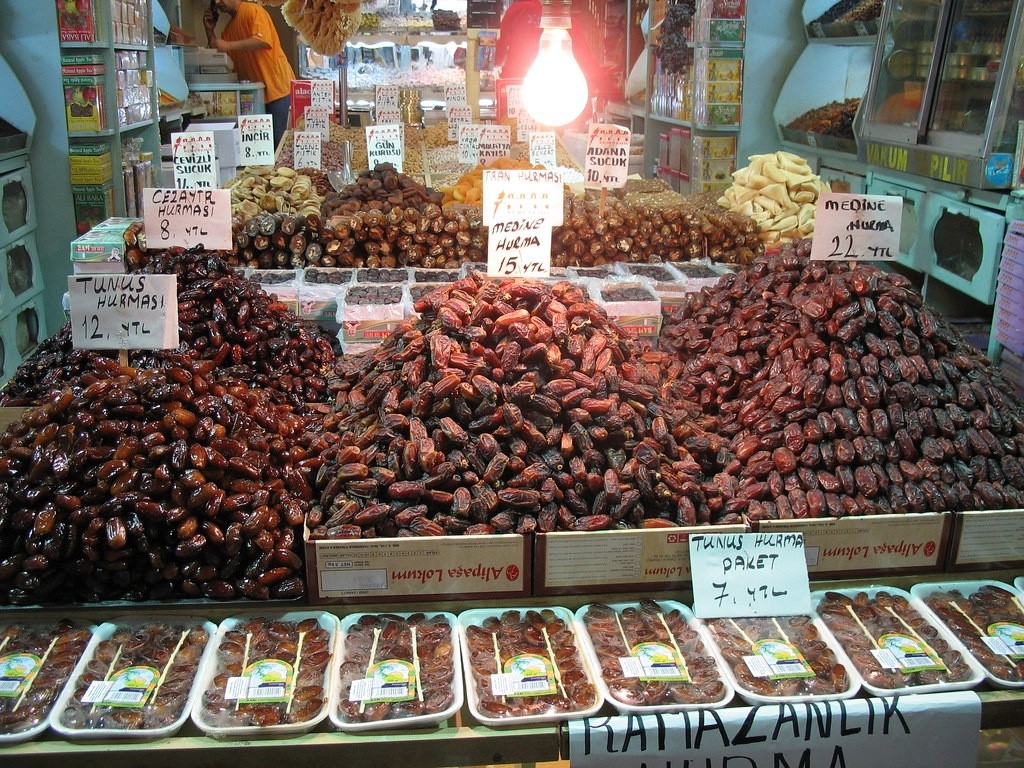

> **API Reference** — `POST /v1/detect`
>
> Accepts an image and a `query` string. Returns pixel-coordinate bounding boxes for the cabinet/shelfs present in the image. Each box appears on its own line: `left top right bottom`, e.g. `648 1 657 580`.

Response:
54 0 164 240
781 147 867 195
772 0 886 183
292 1 749 195
154 44 266 190
862 171 1024 369
0 52 50 397
0 407 1024 768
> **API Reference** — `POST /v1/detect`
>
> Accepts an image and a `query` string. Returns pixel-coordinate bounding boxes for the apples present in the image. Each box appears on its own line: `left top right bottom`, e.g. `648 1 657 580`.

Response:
64 0 91 15
72 86 95 103
77 221 91 234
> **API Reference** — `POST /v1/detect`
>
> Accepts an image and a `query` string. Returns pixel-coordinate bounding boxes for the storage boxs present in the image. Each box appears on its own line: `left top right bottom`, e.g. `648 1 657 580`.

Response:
93 217 144 230
140 151 153 162
61 55 104 65
134 162 146 218
680 128 690 176
101 85 106 128
669 127 687 172
70 161 112 175
533 514 746 598
302 510 532 606
63 75 105 86
670 170 680 194
692 157 735 183
806 0 884 38
106 190 114 218
57 0 95 43
74 262 128 274
70 231 132 263
778 102 858 152
69 151 111 166
679 172 689 196
692 178 733 195
659 132 670 165
98 86 104 129
62 64 105 76
68 141 111 156
945 509 1024 573
693 136 736 160
63 310 71 322
64 86 102 131
62 291 71 310
142 161 152 188
70 170 113 185
167 30 195 46
72 179 113 193
74 192 108 238
124 166 137 218
233 259 741 356
743 509 954 581
660 165 670 183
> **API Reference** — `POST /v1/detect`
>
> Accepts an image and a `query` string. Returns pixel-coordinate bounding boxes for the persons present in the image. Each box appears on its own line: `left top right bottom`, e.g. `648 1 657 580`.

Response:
203 0 296 153
495 0 545 122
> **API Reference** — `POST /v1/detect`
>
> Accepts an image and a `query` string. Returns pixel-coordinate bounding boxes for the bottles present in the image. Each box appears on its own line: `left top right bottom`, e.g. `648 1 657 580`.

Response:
971 21 1008 54
